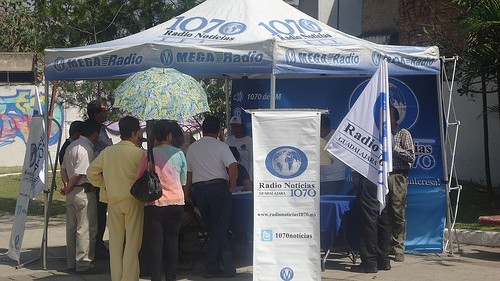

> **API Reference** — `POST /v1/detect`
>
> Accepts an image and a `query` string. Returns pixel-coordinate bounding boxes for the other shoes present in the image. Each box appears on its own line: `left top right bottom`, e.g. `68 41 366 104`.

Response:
394 253 403 261
226 268 236 277
68 268 75 272
77 265 104 274
96 241 108 251
204 268 224 278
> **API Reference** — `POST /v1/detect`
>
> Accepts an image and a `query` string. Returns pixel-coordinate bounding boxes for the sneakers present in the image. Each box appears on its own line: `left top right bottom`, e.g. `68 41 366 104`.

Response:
351 260 378 273
378 259 391 270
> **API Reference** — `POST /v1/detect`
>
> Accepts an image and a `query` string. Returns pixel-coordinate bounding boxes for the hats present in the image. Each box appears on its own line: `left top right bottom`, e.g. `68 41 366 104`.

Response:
229 116 245 126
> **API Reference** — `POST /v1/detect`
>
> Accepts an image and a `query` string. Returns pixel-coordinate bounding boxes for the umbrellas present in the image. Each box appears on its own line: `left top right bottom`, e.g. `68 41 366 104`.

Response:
112 67 211 122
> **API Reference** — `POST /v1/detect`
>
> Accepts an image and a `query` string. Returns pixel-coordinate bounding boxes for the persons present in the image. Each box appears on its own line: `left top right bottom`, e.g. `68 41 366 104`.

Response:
59 100 415 281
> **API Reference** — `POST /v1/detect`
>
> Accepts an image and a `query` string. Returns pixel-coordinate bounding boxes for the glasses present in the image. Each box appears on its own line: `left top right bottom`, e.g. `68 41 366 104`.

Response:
97 109 106 113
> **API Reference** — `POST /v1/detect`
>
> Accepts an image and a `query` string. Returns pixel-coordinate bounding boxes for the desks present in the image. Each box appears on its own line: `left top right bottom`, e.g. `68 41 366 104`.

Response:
232 191 360 271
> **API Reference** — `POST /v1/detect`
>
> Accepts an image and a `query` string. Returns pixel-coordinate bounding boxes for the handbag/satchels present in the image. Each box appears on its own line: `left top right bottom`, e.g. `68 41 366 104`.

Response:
130 147 163 203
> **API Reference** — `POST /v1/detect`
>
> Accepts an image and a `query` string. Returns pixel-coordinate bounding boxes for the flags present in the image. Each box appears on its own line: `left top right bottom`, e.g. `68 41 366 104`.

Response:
324 58 393 215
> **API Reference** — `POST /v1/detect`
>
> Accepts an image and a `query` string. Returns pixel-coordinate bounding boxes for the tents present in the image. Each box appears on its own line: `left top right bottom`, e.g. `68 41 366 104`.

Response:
42 0 462 270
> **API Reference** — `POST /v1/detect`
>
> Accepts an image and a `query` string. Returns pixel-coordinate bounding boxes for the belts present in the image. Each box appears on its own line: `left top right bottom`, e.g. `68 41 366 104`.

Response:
192 179 226 188
